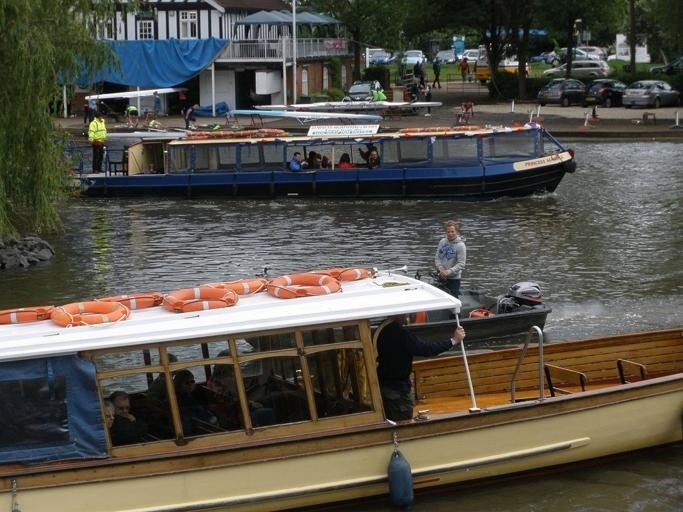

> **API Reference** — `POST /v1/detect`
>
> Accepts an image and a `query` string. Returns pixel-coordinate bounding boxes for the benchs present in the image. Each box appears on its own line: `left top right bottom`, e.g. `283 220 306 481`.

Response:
111 385 263 446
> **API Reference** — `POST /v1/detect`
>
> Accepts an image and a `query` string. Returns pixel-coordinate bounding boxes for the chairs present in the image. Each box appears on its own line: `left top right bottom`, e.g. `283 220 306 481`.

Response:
106 150 127 176
101 102 127 123
455 102 473 125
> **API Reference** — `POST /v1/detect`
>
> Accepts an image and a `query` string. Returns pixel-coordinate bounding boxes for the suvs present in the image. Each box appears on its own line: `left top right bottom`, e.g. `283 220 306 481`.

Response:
541 60 609 80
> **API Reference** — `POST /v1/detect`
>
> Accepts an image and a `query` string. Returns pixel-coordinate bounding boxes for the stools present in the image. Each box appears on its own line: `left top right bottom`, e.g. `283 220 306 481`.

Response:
643 112 656 125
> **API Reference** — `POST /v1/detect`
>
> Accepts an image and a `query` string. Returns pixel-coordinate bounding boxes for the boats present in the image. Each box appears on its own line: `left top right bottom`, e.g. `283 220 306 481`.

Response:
64 120 580 203
241 279 551 354
1 263 683 510
250 99 443 112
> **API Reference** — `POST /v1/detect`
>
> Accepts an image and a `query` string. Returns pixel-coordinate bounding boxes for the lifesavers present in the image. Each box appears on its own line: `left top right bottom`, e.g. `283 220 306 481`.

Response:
306 268 368 281
200 280 270 295
163 287 240 312
0 305 55 323
186 128 290 141
399 125 479 133
91 293 163 310
267 274 342 298
51 301 130 326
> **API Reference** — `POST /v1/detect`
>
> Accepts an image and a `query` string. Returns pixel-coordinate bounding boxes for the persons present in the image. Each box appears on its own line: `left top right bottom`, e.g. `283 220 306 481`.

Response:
411 56 470 117
100 351 281 444
376 312 466 422
289 143 381 171
182 103 198 127
82 89 161 172
435 220 466 299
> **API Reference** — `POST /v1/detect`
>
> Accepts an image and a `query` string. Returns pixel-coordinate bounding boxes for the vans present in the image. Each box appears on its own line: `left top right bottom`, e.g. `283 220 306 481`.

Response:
475 43 529 87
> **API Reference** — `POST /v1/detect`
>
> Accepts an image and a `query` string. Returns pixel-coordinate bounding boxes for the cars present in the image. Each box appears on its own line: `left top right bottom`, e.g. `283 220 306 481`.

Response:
621 80 682 109
581 78 628 108
341 77 384 114
433 49 479 65
536 77 589 107
648 56 683 74
401 50 428 65
530 45 608 67
362 48 399 65
